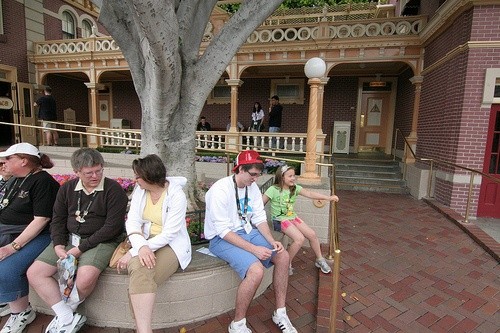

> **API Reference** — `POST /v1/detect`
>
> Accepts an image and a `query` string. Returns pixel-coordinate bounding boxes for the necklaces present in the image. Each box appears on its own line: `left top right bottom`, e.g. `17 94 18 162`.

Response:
75 189 97 230
0 166 40 210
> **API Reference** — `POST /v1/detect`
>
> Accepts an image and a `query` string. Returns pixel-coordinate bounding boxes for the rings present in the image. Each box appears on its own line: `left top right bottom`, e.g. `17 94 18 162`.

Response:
119 266 123 268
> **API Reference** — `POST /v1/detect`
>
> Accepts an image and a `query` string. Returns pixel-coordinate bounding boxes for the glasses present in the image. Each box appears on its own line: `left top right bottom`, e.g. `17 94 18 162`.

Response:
0 162 5 167
133 175 141 182
5 156 18 160
79 167 104 178
246 169 264 177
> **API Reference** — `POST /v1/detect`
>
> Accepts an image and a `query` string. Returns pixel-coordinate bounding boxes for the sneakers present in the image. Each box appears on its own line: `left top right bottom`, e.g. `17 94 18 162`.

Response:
54 311 87 333
271 309 298 333
288 265 294 276
314 256 332 274
44 295 80 333
227 317 252 333
0 301 36 333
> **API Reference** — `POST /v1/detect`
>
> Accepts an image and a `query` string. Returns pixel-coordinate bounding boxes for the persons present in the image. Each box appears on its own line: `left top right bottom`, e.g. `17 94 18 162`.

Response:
0 151 13 196
0 142 60 333
34 87 59 146
263 164 339 275
268 95 282 150
116 154 192 333
198 118 211 146
204 150 298 333
226 117 244 132
27 148 128 333
251 101 264 145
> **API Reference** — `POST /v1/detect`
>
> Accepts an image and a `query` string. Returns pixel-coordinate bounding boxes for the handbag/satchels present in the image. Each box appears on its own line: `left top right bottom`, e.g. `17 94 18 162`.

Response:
109 232 146 268
55 254 77 303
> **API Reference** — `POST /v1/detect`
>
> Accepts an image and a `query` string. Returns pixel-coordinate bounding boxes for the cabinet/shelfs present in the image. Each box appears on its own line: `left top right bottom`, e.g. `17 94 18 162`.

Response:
331 120 351 156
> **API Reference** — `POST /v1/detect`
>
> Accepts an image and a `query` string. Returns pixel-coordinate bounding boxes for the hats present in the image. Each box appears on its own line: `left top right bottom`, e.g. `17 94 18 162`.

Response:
231 150 263 173
0 143 41 158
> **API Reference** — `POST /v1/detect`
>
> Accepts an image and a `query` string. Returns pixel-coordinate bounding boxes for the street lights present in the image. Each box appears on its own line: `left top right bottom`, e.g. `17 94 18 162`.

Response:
295 57 327 186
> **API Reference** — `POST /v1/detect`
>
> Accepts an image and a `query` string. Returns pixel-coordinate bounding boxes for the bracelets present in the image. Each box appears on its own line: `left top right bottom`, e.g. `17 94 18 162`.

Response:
11 242 21 251
